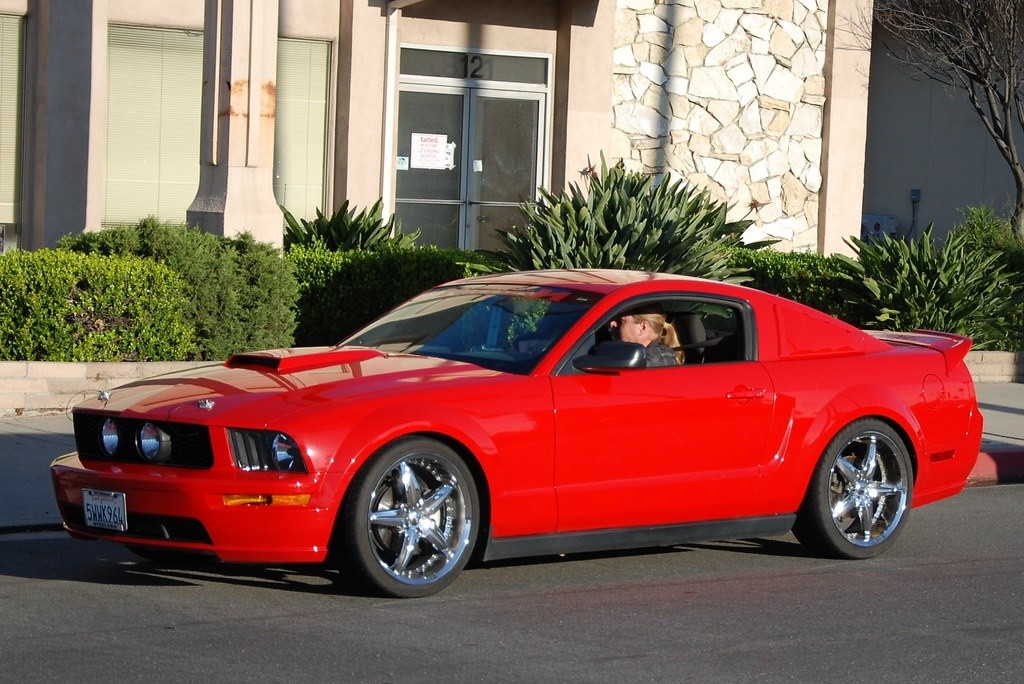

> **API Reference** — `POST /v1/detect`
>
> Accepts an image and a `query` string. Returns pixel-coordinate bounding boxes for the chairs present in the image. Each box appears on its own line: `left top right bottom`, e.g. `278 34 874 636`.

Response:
519 301 611 376
663 310 707 364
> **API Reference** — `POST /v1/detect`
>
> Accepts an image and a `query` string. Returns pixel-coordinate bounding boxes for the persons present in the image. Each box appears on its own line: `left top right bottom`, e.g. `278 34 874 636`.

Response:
610 305 685 367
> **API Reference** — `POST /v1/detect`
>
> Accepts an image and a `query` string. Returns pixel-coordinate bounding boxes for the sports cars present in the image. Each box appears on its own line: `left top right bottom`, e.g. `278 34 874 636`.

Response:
49 270 986 602
